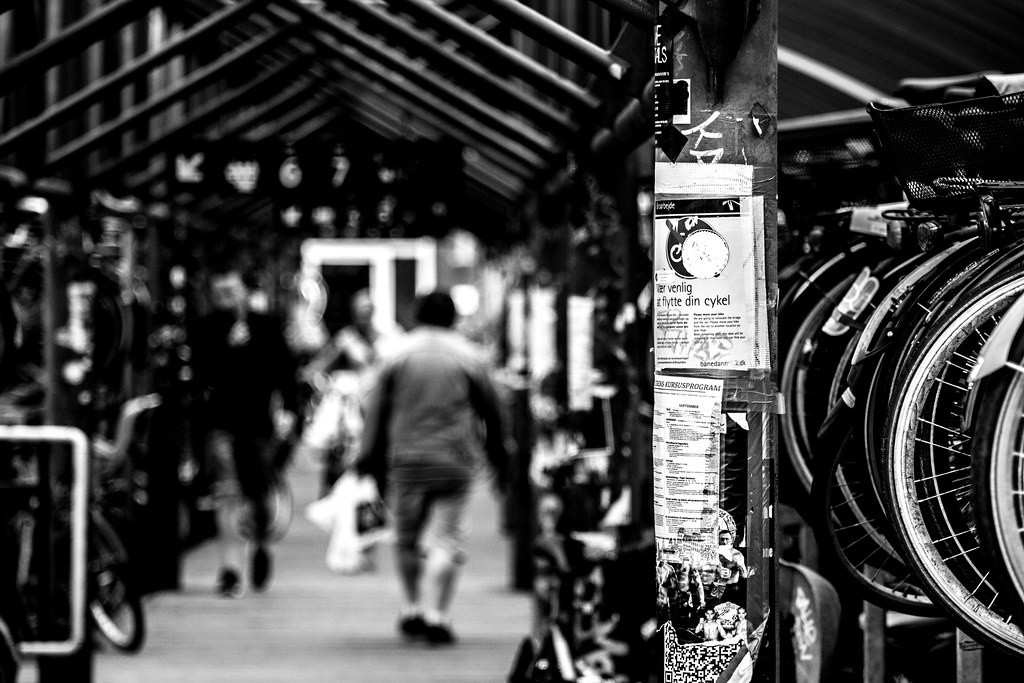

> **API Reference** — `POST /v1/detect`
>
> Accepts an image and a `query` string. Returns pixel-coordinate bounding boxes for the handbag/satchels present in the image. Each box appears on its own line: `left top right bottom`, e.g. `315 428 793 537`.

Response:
306 472 380 573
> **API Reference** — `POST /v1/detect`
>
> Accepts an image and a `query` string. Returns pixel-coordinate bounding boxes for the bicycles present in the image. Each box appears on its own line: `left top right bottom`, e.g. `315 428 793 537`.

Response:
776 68 1024 664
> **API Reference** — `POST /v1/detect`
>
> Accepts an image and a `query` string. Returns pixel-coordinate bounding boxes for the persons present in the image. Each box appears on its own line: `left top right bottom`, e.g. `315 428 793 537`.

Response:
186 271 514 645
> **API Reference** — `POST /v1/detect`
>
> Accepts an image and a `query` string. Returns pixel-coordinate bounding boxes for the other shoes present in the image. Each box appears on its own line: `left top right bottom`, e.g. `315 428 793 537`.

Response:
401 615 427 641
250 548 270 590
424 623 456 645
221 568 245 599
